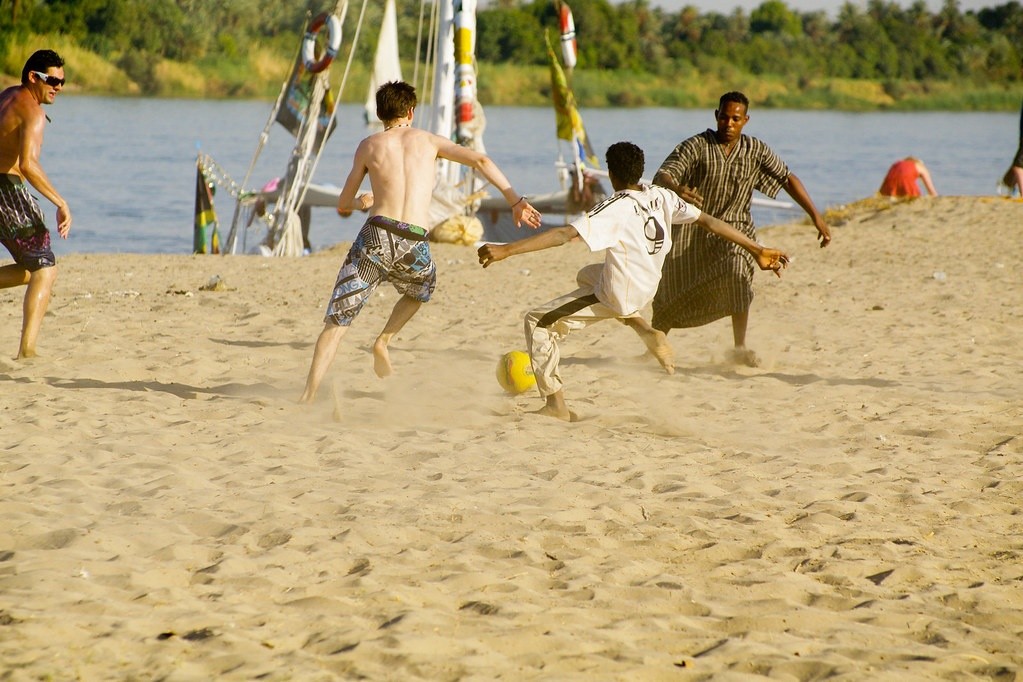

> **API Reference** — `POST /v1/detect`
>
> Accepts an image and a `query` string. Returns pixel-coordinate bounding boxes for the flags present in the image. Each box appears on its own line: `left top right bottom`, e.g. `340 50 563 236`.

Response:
542 29 601 172
273 13 338 156
192 148 220 254
363 0 404 126
430 0 491 215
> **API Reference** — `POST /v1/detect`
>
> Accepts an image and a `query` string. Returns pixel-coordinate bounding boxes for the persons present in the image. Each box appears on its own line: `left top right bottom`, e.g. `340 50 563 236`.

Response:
651 91 832 370
297 78 543 408
0 48 70 362
477 142 790 424
878 153 937 199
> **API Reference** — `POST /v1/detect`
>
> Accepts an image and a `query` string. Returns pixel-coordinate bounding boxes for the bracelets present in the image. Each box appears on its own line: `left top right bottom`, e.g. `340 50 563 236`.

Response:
357 195 368 210
510 196 528 209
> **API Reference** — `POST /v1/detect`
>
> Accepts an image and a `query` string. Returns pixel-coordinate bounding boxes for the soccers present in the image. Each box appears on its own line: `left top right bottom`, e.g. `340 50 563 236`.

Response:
496 352 538 394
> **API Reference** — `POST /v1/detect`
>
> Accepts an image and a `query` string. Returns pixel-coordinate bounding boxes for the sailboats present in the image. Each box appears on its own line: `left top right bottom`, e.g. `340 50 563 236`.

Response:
189 0 613 256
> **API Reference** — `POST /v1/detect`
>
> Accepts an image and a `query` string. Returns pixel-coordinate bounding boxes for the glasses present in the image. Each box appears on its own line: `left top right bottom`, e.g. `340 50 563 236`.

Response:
33 71 65 87
